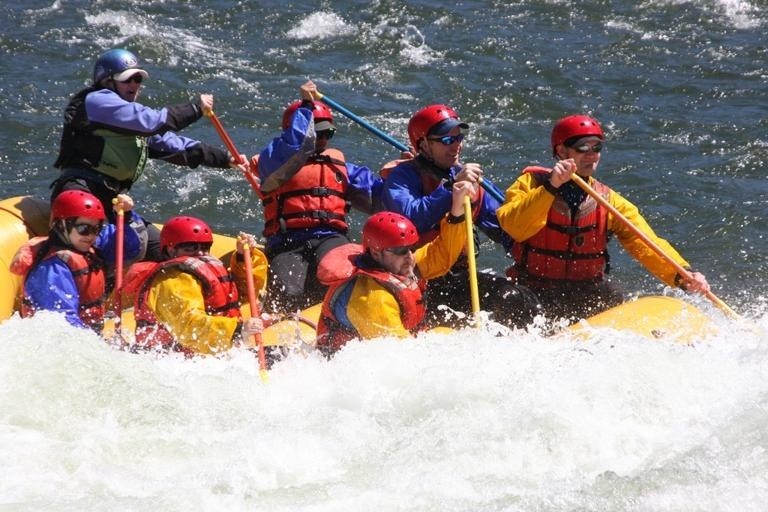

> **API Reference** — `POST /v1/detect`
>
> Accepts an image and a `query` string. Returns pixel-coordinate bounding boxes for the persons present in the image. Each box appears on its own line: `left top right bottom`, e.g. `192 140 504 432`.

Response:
54 43 251 225
258 78 420 308
131 213 265 353
316 178 480 357
16 191 150 339
491 110 712 331
378 101 522 310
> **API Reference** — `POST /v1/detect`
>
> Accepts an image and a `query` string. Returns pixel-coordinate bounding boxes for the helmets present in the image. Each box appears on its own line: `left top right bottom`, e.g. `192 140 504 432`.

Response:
362 210 420 255
159 214 214 259
283 99 338 135
551 115 605 148
93 49 149 91
407 103 469 154
49 189 105 232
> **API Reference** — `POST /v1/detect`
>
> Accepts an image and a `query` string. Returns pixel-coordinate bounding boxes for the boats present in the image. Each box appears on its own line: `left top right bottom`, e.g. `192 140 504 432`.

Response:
0 191 724 378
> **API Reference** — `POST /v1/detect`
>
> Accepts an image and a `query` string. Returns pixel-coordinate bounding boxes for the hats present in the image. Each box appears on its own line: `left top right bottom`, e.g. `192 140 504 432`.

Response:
564 135 605 147
314 119 336 131
109 67 148 82
430 118 468 135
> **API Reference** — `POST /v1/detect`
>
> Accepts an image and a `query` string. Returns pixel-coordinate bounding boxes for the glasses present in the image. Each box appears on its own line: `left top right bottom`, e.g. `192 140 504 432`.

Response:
176 244 210 254
67 222 103 235
427 133 464 145
312 129 334 140
571 144 602 153
384 246 416 256
118 74 142 86
